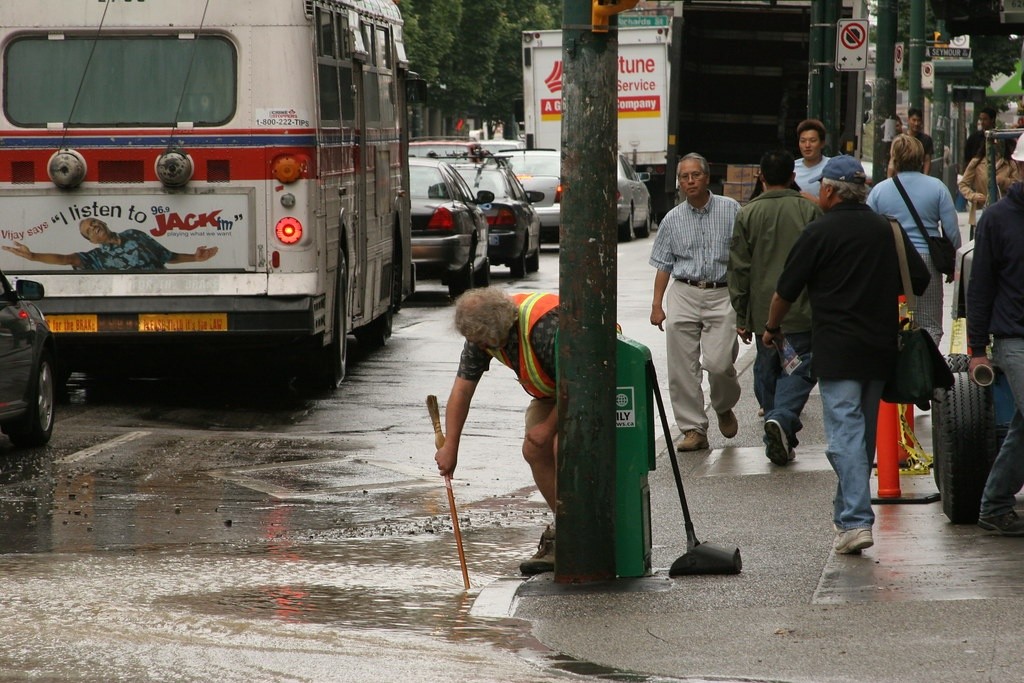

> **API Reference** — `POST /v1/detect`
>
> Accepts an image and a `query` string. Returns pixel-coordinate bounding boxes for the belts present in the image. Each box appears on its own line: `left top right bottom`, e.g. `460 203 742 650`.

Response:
676 278 726 289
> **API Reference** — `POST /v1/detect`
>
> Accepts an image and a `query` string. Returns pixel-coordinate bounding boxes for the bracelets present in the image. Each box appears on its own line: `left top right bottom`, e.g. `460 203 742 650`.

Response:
766 322 781 334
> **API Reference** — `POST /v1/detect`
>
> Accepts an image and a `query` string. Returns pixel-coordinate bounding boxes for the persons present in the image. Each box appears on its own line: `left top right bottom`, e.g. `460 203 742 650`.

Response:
793 119 832 197
907 107 934 175
762 154 932 553
896 116 902 135
964 109 997 168
649 152 743 451
434 286 623 571
958 133 1024 535
726 148 826 466
866 134 962 412
1 218 219 270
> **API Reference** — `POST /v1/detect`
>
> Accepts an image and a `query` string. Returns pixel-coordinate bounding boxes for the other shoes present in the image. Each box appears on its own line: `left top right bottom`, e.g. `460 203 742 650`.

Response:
789 449 795 460
763 419 788 465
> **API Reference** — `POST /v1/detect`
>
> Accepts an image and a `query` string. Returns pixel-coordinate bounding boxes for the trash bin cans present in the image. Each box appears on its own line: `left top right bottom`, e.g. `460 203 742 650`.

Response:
555 324 657 580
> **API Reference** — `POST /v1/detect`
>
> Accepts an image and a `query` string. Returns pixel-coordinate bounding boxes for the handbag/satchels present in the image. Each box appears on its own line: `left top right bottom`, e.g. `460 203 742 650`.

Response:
926 236 956 272
879 318 954 411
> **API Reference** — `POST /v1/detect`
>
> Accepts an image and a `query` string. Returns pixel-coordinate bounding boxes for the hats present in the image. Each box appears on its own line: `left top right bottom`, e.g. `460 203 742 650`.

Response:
808 153 865 185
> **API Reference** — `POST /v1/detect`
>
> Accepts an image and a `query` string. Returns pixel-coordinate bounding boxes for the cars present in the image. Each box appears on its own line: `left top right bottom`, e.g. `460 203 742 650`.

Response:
408 156 496 298
0 266 61 446
408 137 651 279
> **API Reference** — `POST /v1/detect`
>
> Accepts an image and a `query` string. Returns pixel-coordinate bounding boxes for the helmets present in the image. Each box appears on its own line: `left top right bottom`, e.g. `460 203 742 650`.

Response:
1011 133 1024 161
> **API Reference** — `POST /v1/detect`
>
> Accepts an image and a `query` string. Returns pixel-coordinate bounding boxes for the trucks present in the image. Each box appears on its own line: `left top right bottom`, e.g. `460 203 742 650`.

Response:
663 0 868 223
521 27 673 225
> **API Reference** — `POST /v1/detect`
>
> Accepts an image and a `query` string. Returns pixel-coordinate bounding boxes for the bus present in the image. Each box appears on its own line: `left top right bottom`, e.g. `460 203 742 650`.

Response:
1 0 430 392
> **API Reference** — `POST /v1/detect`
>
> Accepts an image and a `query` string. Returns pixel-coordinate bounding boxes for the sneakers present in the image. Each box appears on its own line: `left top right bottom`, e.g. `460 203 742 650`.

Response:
977 512 1024 535
677 430 709 451
519 529 557 573
834 528 873 554
717 408 738 438
834 524 850 538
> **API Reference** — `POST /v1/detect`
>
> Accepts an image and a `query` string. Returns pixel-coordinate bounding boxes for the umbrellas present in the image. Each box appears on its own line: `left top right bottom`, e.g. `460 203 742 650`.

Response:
985 58 1024 96
